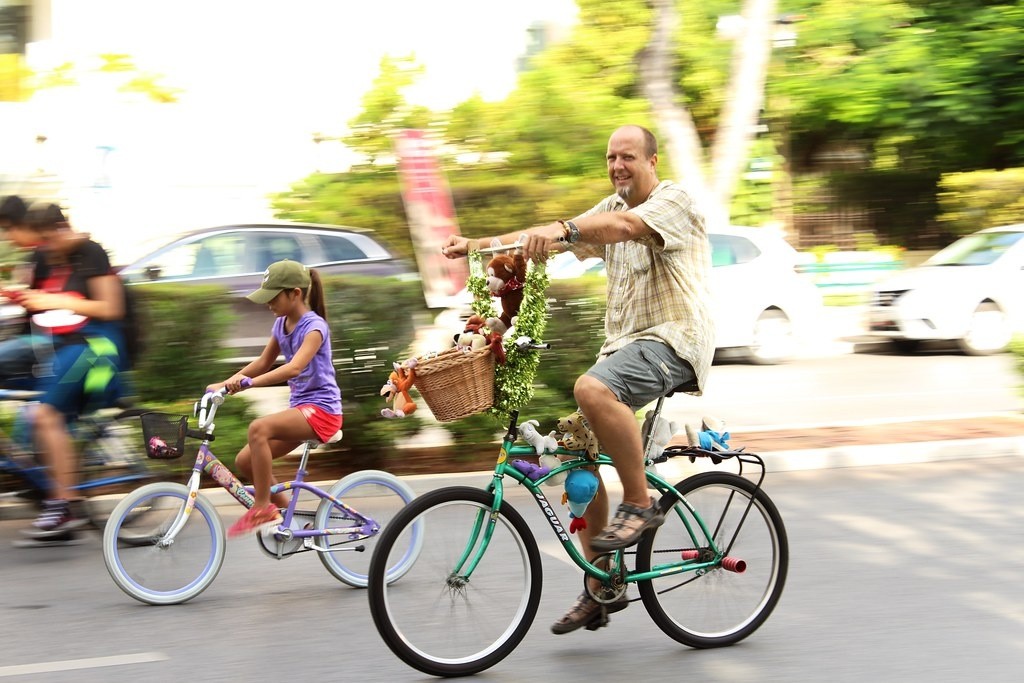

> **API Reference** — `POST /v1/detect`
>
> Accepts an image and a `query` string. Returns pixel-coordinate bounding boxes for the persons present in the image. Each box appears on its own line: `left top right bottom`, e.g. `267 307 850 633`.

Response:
203 258 343 540
0 195 142 548
442 124 717 633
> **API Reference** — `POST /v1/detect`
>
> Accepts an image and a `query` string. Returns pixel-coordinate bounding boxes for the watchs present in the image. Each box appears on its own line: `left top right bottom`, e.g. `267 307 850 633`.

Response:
566 221 579 243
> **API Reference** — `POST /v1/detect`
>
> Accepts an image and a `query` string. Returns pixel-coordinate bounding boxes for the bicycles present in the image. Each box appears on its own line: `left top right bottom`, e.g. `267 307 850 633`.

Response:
368 237 788 677
0 381 198 545
102 377 424 605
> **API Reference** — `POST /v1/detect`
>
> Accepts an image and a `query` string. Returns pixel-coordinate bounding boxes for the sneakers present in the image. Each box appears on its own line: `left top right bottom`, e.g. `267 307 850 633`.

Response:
22 498 89 538
551 591 628 635
304 521 313 529
227 502 284 535
590 497 665 548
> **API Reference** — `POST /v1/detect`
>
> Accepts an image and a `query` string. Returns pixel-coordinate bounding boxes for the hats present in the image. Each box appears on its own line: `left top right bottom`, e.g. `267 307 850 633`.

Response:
247 256 310 304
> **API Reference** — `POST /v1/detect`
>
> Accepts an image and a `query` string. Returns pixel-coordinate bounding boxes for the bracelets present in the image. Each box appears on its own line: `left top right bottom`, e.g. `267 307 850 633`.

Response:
558 220 571 243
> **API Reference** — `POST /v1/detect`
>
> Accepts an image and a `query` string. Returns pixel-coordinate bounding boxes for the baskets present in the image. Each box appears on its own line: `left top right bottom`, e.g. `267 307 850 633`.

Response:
408 344 496 422
141 412 189 458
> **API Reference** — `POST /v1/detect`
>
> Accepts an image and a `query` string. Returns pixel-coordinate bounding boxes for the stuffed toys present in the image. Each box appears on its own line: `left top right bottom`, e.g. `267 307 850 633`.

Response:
508 412 601 534
640 410 679 463
377 358 418 420
685 415 730 467
452 234 525 365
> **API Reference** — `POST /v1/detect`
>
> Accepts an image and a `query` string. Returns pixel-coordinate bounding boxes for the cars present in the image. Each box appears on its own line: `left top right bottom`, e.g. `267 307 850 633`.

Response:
112 222 446 403
861 224 1024 356
580 226 822 365
